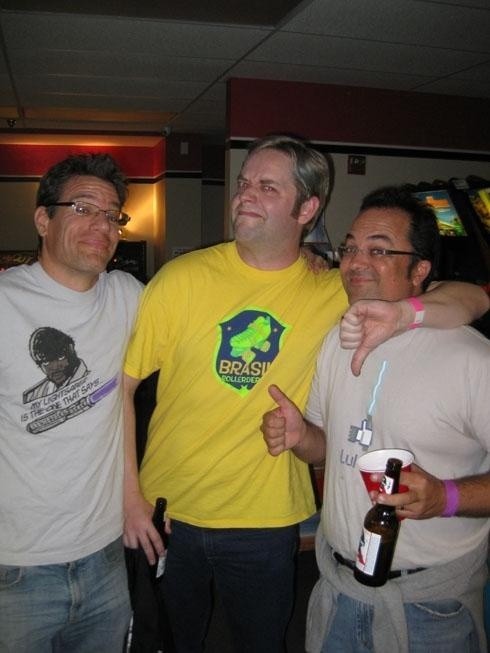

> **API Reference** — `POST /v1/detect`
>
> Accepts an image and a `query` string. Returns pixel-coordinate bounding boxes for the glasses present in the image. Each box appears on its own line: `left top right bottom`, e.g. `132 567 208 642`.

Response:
339 246 418 256
54 201 130 225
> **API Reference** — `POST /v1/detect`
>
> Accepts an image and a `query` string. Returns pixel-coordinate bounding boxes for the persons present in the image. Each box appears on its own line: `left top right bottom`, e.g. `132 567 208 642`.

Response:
0 152 329 652
118 132 489 652
260 185 489 653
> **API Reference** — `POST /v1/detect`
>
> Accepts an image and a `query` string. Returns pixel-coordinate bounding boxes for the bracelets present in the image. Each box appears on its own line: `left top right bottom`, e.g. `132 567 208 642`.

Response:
441 480 459 518
407 296 424 330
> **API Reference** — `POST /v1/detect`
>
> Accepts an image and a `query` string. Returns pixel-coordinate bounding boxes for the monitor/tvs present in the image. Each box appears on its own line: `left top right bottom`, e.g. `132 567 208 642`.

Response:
468 188 490 233
410 189 468 237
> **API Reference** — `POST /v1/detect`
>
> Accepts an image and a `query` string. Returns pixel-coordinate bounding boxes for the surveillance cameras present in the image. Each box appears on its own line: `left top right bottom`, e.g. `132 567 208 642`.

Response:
161 125 172 136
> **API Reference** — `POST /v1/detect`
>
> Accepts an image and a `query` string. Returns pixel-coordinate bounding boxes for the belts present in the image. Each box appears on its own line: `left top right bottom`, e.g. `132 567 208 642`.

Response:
333 552 424 579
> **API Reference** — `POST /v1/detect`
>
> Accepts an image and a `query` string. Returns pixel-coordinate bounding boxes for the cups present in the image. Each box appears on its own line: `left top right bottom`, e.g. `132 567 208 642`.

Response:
354 448 416 520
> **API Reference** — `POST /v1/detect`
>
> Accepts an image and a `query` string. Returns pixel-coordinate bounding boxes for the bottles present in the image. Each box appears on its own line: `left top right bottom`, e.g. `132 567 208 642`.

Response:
136 496 172 582
352 457 403 590
